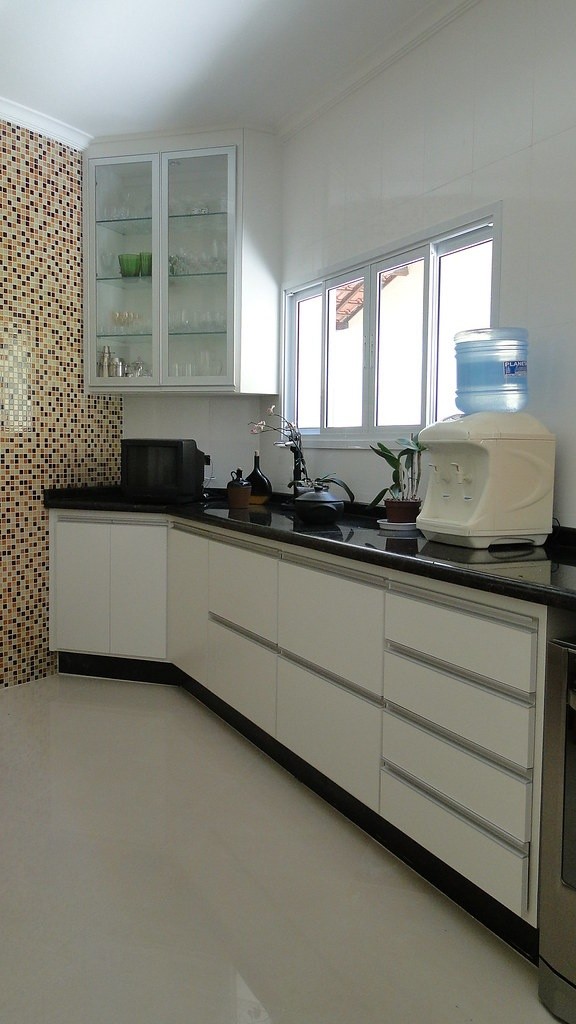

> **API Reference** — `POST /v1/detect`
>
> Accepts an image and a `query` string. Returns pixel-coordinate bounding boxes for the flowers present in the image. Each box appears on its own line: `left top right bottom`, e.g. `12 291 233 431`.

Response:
247 404 355 504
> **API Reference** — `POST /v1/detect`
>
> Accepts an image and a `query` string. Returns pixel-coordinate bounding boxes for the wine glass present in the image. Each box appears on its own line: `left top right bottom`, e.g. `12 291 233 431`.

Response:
113 312 139 334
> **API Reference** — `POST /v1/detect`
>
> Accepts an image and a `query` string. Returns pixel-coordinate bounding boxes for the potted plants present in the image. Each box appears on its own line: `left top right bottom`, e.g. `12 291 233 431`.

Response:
365 432 427 523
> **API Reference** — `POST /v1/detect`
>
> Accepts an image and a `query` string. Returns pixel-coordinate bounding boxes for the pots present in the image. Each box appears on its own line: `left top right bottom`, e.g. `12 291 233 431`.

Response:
282 486 344 524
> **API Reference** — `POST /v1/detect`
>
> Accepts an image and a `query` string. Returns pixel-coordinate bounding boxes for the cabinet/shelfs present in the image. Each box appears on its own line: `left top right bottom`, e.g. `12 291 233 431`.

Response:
38 486 576 968
81 124 281 396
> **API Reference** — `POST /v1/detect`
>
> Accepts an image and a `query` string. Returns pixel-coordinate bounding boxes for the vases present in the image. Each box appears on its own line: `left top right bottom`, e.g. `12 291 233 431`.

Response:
296 486 329 496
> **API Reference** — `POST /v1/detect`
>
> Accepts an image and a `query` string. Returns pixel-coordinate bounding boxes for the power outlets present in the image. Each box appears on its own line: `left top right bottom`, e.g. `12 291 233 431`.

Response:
204 449 214 477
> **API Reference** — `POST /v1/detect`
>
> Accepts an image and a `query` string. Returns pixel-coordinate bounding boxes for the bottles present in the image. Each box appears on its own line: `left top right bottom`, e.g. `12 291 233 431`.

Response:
453 327 529 414
245 450 272 504
226 468 252 509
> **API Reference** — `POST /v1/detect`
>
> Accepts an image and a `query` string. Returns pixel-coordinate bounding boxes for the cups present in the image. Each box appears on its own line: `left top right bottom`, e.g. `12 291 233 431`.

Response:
169 350 222 376
170 309 228 331
118 254 139 277
140 252 152 276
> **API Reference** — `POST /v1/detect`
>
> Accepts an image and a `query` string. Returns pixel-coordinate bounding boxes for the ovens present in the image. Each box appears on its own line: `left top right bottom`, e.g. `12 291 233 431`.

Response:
536 640 576 1024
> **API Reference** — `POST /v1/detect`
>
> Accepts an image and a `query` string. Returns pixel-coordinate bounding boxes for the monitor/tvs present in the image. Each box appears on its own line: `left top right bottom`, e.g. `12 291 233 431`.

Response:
119 438 205 501
